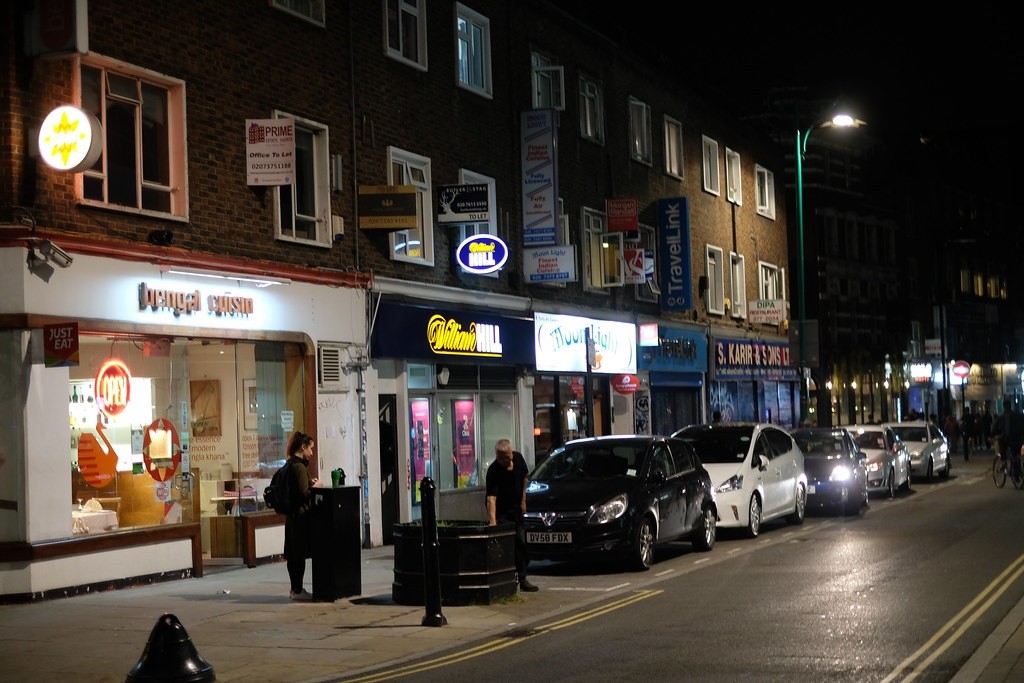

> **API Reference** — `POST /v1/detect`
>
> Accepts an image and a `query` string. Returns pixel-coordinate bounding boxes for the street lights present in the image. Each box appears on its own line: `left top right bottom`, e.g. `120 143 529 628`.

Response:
797 112 866 427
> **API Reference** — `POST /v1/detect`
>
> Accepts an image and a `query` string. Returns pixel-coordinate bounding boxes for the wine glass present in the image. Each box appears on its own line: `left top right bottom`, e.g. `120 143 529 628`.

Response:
77 499 83 513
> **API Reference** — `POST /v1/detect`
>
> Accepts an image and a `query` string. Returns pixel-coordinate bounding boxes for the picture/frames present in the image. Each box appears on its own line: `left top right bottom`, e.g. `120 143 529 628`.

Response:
242 378 258 430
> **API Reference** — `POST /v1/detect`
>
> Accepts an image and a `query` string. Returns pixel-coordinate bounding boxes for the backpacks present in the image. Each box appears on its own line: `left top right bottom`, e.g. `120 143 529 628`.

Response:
264 461 309 514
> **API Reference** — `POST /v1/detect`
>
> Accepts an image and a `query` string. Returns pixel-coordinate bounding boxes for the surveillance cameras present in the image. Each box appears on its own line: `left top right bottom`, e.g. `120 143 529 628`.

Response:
38 237 74 268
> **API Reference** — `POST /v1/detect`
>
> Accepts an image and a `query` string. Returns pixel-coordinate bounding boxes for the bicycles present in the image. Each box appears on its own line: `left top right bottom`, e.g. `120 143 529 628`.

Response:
989 437 1024 491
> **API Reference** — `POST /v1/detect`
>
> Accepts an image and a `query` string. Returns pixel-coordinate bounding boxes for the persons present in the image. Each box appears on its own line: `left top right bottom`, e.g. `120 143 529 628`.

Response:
988 401 1024 483
485 439 540 592
867 414 876 424
960 407 992 461
903 409 937 422
944 417 960 450
278 432 323 600
713 412 721 423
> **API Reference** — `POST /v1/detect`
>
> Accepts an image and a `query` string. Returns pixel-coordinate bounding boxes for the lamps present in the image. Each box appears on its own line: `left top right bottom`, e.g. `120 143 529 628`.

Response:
28 238 74 270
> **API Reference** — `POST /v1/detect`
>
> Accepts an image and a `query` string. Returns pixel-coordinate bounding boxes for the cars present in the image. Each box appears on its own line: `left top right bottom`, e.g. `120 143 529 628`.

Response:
781 425 870 518
650 421 808 539
525 434 718 573
826 424 913 499
873 421 951 483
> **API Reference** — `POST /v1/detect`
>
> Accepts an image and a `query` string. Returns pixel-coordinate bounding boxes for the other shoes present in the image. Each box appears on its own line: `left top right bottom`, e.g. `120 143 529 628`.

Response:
520 581 538 591
289 589 312 601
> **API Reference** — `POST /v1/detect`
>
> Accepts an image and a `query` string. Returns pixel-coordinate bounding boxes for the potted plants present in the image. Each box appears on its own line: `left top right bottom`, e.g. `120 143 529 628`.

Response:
391 518 516 605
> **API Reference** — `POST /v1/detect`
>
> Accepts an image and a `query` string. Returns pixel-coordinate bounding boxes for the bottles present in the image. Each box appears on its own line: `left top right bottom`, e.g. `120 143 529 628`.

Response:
220 463 232 481
69 384 108 447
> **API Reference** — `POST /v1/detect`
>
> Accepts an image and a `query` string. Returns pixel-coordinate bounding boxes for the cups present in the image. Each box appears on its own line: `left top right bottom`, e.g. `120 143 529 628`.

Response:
331 470 340 488
